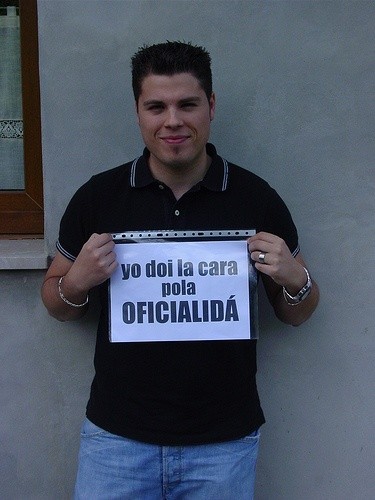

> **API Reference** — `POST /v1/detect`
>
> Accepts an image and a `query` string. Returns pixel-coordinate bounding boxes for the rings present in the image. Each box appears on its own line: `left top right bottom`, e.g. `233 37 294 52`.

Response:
258 251 267 264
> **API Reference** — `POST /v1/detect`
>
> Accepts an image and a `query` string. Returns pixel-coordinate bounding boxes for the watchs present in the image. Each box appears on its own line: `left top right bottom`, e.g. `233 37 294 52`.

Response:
282 266 313 306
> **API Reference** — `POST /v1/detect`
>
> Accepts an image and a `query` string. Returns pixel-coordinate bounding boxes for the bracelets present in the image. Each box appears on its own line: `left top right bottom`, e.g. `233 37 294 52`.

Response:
58 276 89 307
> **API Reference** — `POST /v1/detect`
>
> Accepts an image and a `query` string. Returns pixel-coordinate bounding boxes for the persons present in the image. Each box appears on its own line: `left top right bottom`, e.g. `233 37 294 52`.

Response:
42 40 320 500
